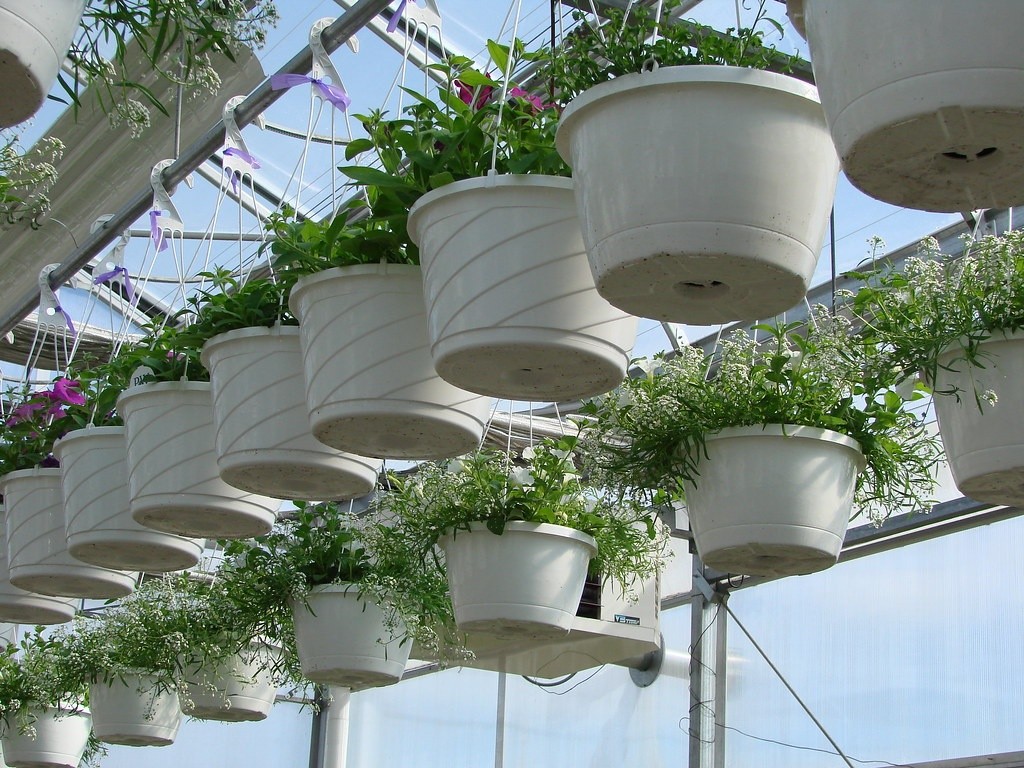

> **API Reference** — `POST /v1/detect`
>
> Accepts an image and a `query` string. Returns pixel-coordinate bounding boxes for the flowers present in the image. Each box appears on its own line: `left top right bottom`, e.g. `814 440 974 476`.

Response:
159 577 284 714
60 606 177 717
250 504 443 671
845 224 1024 408
48 0 288 140
585 305 939 529
363 41 580 225
5 378 87 469
0 625 108 768
0 115 66 231
395 449 668 603
159 350 187 374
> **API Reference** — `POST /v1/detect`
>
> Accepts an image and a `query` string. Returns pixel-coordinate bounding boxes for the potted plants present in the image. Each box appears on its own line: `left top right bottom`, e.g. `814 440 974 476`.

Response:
270 199 494 460
0 461 81 624
115 315 280 540
544 10 838 326
194 258 382 502
49 345 206 574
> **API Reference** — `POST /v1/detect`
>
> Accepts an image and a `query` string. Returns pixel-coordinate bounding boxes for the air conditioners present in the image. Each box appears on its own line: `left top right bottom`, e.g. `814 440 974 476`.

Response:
407 484 659 678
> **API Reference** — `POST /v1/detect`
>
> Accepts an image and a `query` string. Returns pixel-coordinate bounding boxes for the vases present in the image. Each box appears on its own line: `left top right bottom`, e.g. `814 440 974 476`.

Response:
175 632 289 723
3 468 141 600
290 584 415 689
406 174 634 404
917 326 1024 506
777 0 1024 214
84 667 181 747
434 520 598 641
0 0 89 128
0 702 92 768
676 422 865 580
